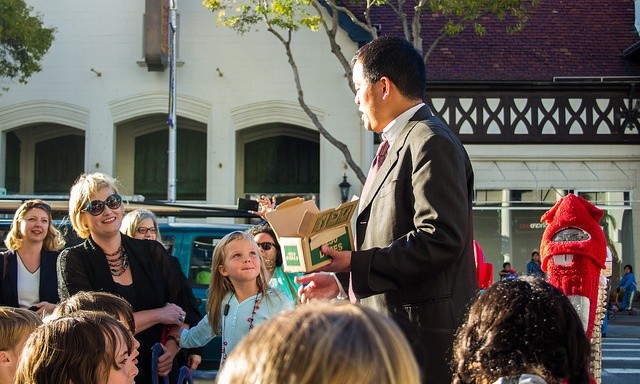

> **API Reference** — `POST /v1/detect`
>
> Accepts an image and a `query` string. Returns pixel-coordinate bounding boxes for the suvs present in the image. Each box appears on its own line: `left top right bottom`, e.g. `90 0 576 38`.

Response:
2 193 265 380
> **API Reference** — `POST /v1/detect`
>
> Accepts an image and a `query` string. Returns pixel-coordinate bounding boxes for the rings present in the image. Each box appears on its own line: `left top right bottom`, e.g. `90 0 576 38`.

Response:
181 315 185 322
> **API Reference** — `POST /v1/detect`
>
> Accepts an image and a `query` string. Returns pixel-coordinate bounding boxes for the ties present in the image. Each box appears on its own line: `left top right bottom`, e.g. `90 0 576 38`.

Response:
358 140 390 217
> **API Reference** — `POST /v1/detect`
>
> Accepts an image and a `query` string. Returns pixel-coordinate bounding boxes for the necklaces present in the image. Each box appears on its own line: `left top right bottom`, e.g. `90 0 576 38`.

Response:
107 257 127 266
111 263 129 276
106 251 125 262
109 261 129 271
104 246 122 257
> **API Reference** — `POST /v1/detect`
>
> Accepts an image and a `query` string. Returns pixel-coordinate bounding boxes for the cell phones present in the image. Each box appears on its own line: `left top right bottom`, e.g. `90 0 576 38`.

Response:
27 305 40 312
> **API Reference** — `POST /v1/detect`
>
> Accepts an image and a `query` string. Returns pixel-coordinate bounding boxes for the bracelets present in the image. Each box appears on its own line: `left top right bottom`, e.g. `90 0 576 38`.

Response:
166 335 180 351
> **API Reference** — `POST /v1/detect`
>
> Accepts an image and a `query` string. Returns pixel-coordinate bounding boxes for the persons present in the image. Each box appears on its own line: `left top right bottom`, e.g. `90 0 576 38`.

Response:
499 262 515 279
244 195 306 306
0 199 66 319
616 264 637 312
166 231 294 369
52 290 141 365
56 172 195 384
446 275 590 384
15 310 139 384
0 306 45 384
526 251 544 278
217 298 421 384
118 208 203 384
294 34 480 383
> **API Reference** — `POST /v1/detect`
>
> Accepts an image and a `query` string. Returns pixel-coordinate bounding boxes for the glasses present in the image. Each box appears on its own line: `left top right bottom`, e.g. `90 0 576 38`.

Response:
81 194 122 216
136 227 158 234
257 242 277 251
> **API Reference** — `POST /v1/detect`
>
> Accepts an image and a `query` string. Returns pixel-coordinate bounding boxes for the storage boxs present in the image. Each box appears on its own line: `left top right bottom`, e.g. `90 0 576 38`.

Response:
263 196 360 274
